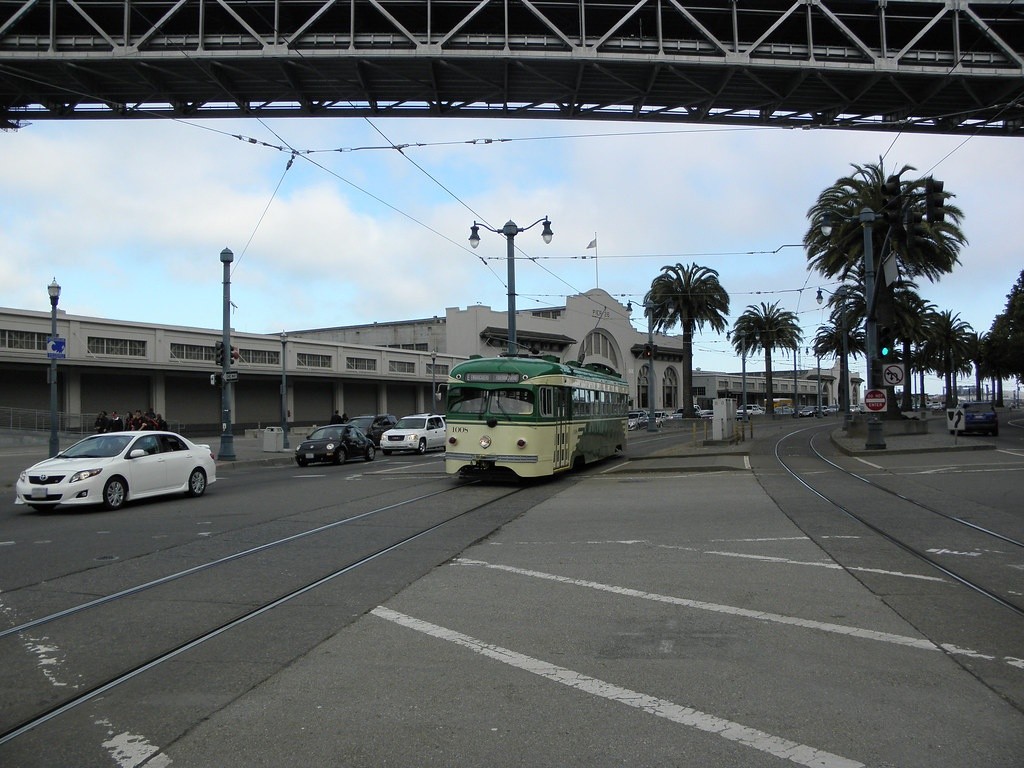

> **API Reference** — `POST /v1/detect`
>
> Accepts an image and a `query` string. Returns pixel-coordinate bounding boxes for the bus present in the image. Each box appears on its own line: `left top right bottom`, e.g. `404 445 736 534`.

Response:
763 398 792 408
435 306 629 486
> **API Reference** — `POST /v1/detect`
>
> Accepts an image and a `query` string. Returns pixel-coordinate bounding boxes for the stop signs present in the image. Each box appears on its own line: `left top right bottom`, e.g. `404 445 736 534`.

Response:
864 389 888 413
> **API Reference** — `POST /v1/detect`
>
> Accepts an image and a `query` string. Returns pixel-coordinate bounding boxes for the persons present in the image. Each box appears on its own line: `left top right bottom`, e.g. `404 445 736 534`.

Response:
96 408 170 434
329 410 349 424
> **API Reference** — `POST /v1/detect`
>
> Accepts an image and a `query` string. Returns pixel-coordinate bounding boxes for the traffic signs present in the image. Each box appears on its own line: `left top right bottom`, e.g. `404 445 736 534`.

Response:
223 371 239 382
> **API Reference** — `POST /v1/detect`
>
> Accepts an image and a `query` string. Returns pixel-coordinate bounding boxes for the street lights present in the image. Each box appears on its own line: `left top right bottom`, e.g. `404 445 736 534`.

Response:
820 206 887 450
625 299 674 432
806 346 823 418
726 327 761 422
816 287 852 431
47 276 63 458
468 214 555 356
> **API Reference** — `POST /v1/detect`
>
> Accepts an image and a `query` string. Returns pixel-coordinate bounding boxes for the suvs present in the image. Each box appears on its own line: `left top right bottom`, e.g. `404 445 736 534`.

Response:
379 413 447 456
345 413 398 447
736 404 761 421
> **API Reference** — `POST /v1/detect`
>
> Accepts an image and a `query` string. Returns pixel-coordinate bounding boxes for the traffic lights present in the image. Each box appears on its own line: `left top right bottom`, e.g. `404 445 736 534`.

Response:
878 324 892 360
906 212 927 250
644 344 652 358
882 175 903 226
926 178 945 224
215 342 224 364
230 344 235 364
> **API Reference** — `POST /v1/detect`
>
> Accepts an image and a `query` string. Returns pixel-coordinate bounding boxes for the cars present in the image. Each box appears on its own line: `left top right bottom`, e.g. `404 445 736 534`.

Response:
816 404 840 416
644 410 674 429
849 405 860 413
672 404 714 420
16 430 217 513
912 401 943 411
296 423 375 467
963 402 998 437
774 406 794 414
799 406 818 418
628 412 646 432
